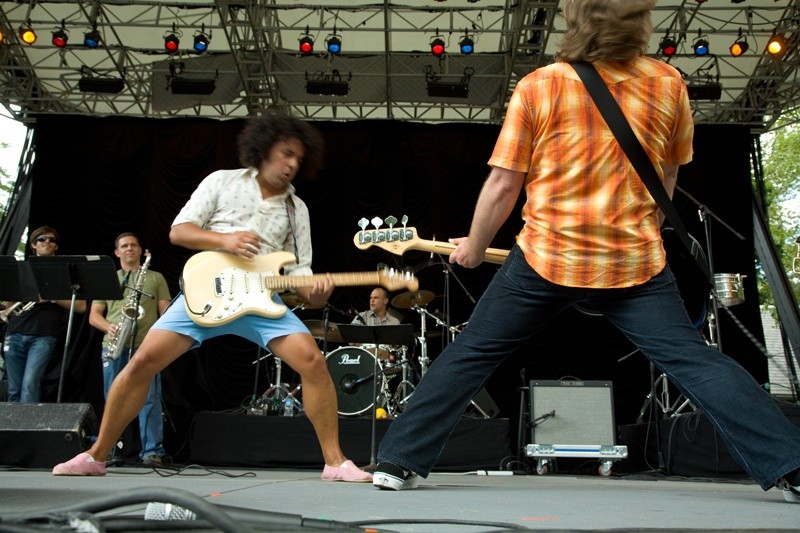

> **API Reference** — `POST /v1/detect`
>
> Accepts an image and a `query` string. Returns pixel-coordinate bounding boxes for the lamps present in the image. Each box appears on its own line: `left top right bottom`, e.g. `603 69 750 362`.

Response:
83 27 100 49
162 22 182 53
52 19 68 47
18 18 37 45
192 22 212 52
458 28 474 54
729 27 749 57
659 28 676 55
296 24 313 53
768 35 785 55
324 26 342 53
691 27 709 57
429 27 445 55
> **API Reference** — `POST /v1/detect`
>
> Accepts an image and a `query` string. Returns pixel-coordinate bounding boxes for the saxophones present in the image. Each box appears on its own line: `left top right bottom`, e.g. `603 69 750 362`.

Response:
106 249 152 362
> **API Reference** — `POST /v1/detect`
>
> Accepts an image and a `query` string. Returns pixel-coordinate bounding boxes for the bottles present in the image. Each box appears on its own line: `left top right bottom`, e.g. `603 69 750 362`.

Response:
284 393 294 416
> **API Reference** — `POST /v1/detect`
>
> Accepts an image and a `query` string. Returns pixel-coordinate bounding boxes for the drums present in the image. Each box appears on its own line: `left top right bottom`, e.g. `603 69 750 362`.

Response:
350 342 391 362
378 348 402 374
712 272 746 307
325 346 388 417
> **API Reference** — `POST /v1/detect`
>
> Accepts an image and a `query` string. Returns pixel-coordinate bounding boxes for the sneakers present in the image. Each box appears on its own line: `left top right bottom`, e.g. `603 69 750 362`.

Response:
783 480 800 503
372 460 417 490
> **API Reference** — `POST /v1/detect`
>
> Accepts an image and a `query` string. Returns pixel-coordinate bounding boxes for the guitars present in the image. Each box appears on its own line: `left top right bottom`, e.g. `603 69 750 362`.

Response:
354 215 709 326
179 251 419 327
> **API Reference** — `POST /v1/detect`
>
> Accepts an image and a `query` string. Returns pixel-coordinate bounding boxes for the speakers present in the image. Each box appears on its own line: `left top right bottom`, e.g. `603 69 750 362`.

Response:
529 379 618 446
462 385 501 419
0 402 98 469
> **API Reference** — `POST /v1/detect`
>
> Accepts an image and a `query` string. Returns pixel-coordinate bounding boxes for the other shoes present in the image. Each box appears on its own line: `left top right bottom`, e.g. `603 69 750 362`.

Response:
143 455 161 467
52 452 107 476
321 459 373 481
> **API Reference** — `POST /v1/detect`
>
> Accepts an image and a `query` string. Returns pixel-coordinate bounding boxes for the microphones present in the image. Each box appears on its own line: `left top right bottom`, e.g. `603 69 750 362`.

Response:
550 410 555 417
122 269 131 293
355 309 367 325
343 381 357 390
431 233 436 258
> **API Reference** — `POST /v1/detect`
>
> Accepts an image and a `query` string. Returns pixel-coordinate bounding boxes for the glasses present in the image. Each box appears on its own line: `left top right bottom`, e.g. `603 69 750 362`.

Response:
34 236 56 243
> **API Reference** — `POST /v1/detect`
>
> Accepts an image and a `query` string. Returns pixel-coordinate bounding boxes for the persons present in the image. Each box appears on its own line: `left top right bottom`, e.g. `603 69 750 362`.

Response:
3 225 64 404
351 288 403 326
371 0 800 504
88 231 175 468
53 108 374 484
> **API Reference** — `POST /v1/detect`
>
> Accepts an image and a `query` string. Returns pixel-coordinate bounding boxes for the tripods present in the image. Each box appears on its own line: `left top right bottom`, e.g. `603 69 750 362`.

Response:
257 356 303 407
390 345 418 416
636 371 698 424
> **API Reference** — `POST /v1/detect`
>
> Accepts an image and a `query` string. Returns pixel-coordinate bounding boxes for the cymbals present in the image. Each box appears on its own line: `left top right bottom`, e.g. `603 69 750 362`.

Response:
281 292 328 310
300 319 344 343
391 290 436 309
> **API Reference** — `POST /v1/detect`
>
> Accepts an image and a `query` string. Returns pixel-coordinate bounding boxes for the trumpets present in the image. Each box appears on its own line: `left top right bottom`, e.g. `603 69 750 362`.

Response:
0 301 37 322
